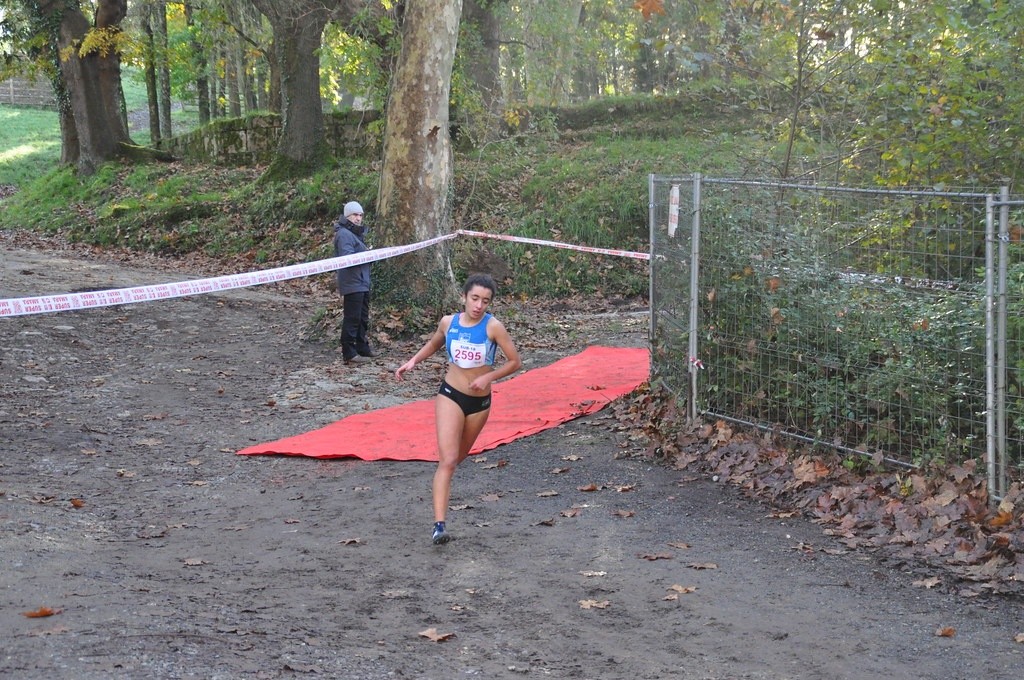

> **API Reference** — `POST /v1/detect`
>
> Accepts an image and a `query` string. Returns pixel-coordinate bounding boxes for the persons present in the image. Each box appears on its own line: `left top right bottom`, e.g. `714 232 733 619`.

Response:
394 272 523 544
333 201 382 365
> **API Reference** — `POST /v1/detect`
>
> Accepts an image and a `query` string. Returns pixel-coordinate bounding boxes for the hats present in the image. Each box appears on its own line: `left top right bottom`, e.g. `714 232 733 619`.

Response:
344 201 364 216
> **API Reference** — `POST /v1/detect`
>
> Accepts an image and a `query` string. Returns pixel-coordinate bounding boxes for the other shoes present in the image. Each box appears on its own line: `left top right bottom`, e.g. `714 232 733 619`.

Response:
362 351 379 358
347 354 371 367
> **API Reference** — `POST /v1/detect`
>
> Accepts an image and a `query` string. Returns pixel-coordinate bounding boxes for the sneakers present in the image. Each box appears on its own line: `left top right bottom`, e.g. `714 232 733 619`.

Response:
432 521 451 545
